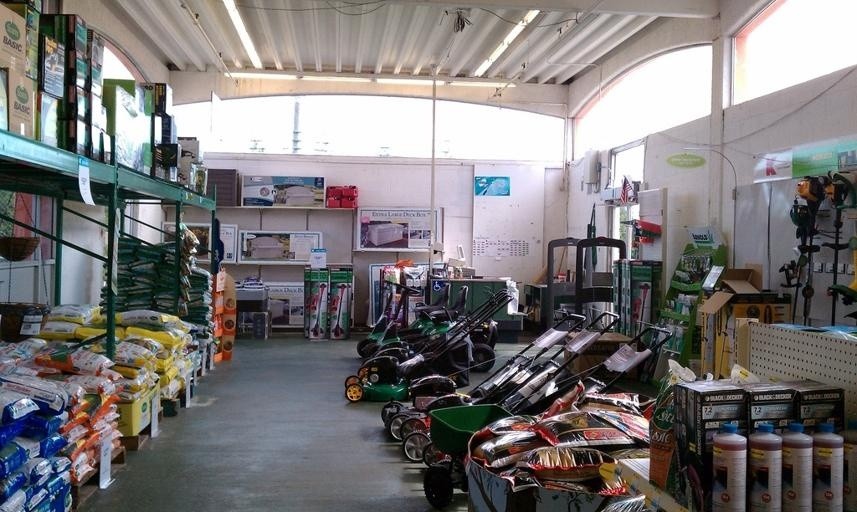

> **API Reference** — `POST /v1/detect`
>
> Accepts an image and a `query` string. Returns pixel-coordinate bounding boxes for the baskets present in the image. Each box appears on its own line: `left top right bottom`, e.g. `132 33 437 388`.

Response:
0 236 41 262
0 301 52 343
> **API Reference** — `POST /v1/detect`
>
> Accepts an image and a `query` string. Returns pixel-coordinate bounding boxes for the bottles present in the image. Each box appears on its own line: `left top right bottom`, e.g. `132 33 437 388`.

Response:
710 423 856 511
189 161 207 196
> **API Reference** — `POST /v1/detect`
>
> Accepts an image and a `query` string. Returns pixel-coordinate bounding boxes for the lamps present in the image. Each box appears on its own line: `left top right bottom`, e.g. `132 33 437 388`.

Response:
666 147 738 270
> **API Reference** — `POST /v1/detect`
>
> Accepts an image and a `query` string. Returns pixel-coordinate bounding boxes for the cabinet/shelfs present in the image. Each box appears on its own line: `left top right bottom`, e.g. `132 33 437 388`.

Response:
747 321 856 431
525 284 576 326
645 241 729 389
163 168 446 341
430 277 517 321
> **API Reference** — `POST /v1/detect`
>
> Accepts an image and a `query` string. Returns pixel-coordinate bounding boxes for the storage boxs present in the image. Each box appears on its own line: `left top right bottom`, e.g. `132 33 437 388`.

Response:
466 415 632 512
611 259 662 336
117 378 162 438
0 0 204 187
699 262 792 378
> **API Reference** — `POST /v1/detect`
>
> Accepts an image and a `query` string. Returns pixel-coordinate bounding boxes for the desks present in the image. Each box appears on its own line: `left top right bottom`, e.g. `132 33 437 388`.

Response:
618 458 691 512
